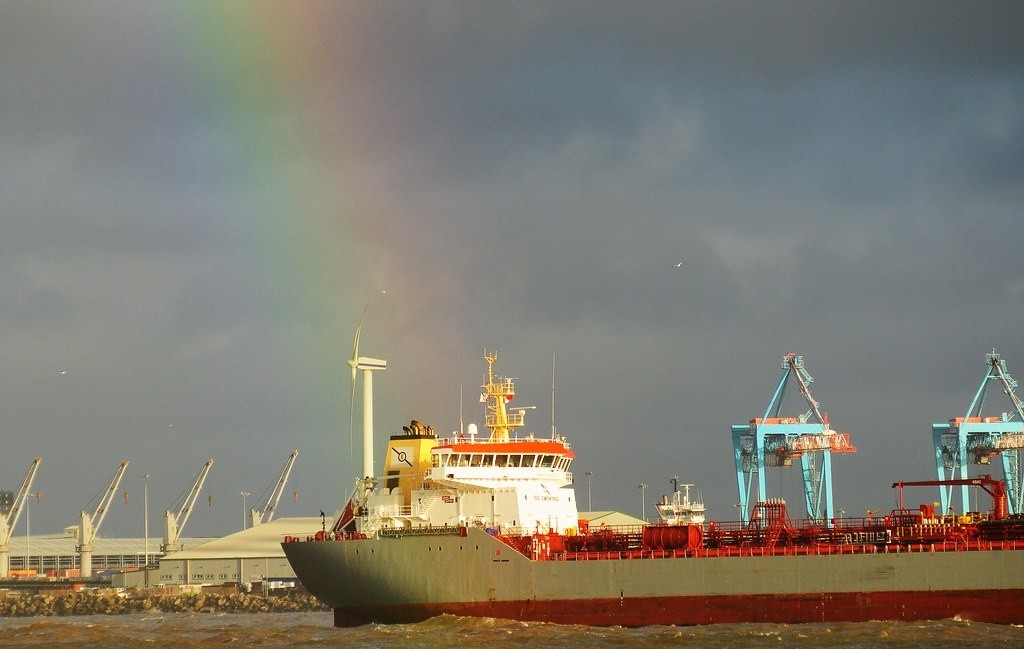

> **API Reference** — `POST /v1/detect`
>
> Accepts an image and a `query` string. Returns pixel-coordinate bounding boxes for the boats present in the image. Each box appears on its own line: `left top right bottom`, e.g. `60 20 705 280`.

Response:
279 347 1024 633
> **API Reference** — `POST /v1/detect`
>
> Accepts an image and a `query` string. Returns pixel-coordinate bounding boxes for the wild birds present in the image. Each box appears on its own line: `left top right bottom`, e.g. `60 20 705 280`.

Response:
672 260 685 270
